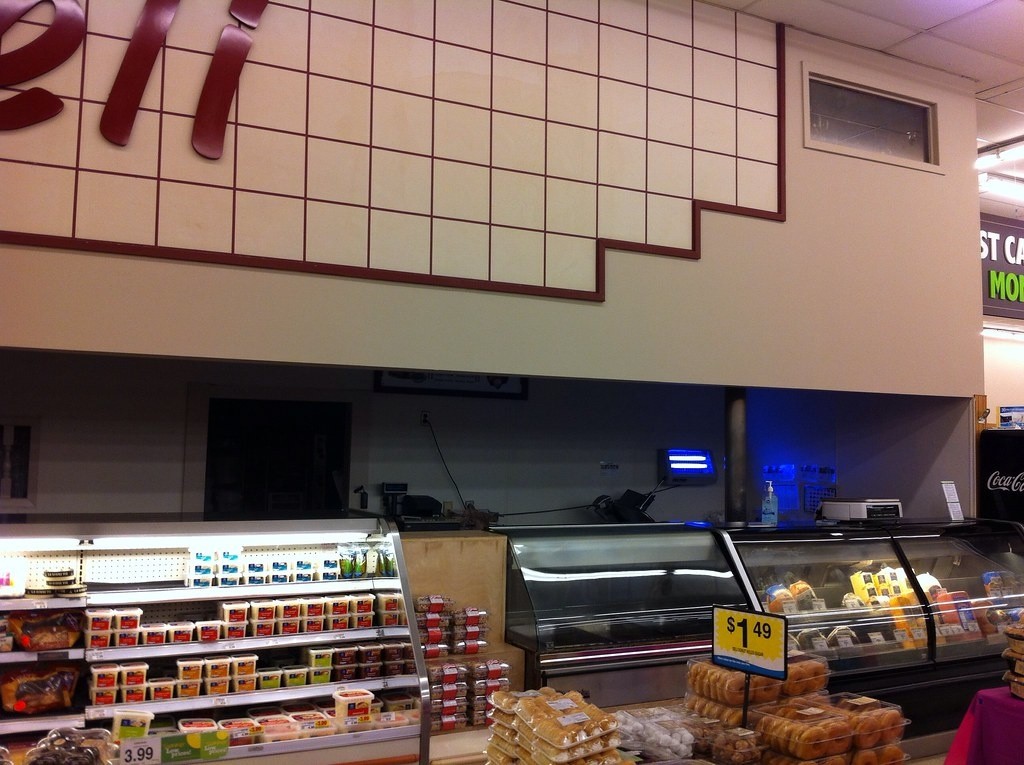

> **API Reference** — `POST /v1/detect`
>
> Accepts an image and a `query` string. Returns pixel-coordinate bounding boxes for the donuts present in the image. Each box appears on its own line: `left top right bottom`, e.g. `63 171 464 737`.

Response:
688 658 906 765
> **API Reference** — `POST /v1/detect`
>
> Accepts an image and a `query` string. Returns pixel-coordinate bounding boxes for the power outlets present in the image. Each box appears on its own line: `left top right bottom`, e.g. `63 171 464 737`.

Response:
443 501 453 515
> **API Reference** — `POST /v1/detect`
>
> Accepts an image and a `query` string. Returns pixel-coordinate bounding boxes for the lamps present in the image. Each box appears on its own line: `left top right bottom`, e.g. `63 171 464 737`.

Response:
658 448 719 487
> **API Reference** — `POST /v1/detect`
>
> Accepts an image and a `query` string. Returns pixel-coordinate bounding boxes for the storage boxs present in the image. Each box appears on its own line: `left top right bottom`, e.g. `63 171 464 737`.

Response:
87 547 910 765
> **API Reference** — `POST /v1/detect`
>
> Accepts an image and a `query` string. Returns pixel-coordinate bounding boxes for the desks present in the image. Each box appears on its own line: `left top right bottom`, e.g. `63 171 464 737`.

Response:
944 686 1024 765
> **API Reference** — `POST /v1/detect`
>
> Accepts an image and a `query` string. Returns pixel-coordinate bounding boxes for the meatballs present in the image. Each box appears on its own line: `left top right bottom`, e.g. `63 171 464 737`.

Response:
612 706 763 765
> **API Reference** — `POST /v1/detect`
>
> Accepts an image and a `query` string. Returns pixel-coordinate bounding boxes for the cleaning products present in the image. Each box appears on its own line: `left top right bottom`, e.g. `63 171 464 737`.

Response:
761 480 778 527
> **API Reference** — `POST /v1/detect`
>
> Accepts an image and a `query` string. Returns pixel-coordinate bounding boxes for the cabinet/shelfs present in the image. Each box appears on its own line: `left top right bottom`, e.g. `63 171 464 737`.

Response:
0 520 431 765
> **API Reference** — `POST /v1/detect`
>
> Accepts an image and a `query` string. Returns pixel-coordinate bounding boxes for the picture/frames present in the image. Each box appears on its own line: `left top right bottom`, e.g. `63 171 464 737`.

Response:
374 369 529 401
